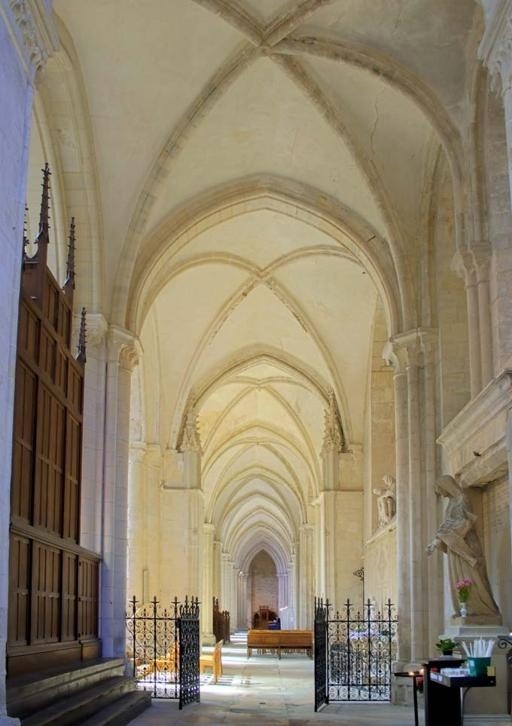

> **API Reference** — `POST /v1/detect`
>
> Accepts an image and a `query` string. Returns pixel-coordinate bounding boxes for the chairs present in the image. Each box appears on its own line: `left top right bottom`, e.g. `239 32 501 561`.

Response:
147 640 226 686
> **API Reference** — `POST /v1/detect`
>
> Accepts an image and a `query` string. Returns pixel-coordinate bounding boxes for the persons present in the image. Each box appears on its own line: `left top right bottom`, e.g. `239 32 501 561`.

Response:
372 473 397 520
424 474 501 619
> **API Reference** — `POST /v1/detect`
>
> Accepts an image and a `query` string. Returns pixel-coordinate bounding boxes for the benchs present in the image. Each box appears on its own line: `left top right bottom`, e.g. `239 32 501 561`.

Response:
245 627 315 659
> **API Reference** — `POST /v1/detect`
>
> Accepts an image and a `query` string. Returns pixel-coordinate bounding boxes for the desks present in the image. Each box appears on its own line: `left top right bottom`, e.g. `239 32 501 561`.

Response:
419 657 496 726
394 668 429 726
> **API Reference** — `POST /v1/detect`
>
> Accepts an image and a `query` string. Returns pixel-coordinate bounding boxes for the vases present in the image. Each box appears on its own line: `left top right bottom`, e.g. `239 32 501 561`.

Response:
458 601 469 619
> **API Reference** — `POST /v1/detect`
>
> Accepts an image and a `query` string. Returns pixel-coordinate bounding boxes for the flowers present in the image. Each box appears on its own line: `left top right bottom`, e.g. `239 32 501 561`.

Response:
454 579 472 604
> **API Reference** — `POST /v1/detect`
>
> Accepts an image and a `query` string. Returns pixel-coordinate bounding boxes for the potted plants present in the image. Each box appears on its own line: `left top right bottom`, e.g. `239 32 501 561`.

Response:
434 638 460 657
